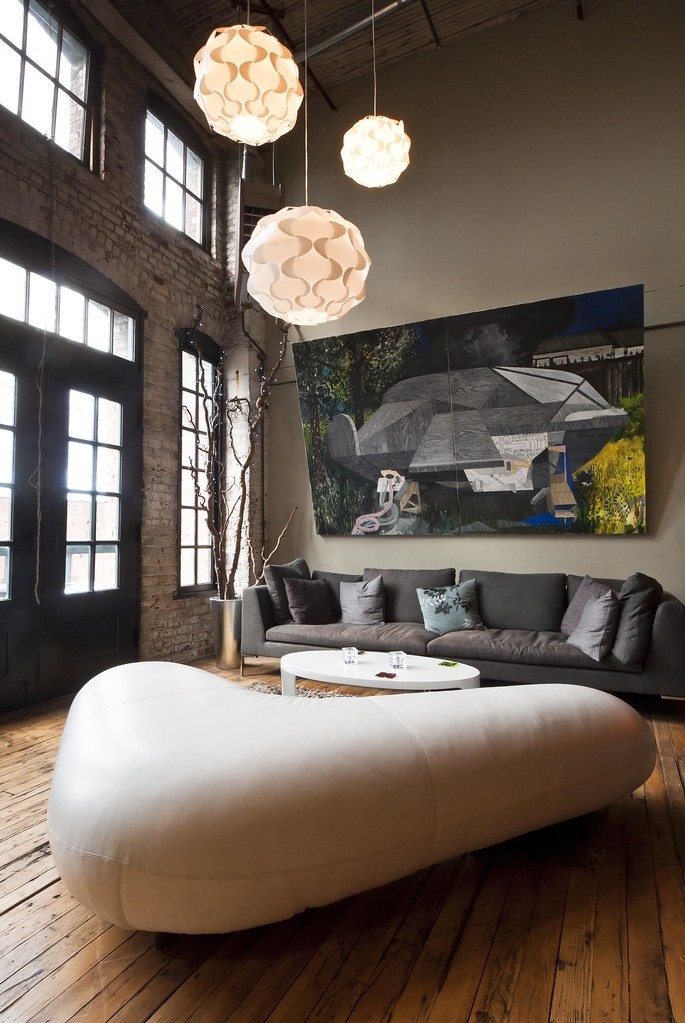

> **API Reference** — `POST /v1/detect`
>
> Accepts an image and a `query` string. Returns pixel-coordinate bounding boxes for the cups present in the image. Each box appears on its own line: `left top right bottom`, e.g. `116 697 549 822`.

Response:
341 647 358 664
388 650 407 669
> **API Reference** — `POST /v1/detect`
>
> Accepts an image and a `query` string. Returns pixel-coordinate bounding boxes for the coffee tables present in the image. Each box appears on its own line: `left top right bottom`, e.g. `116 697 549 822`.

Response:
279 649 480 697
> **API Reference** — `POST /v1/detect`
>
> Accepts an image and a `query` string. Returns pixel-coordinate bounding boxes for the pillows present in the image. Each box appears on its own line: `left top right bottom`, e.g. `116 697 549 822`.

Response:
612 572 663 665
264 557 311 625
337 574 386 626
416 579 486 635
283 576 343 627
561 574 619 637
565 589 619 663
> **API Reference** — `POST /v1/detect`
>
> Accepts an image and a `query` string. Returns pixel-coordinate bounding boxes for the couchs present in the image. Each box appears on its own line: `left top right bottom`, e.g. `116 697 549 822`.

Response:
241 583 685 697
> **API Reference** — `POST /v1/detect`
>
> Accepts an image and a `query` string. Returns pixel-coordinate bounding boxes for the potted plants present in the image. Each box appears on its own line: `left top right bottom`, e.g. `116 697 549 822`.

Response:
181 312 299 674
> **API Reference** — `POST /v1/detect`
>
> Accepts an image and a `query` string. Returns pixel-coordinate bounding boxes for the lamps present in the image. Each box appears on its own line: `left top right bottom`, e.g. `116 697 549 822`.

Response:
186 0 305 146
241 0 372 326
340 0 412 188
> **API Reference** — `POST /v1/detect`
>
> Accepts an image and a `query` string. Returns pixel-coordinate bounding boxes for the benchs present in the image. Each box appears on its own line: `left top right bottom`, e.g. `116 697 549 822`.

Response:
41 660 658 937
